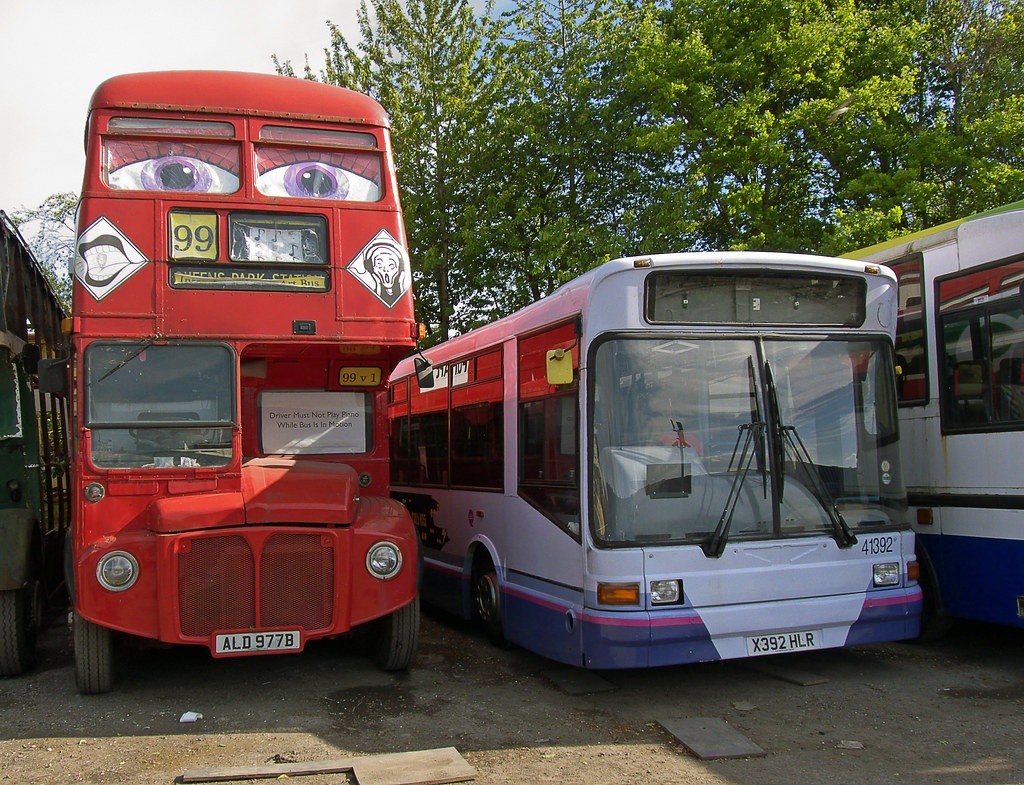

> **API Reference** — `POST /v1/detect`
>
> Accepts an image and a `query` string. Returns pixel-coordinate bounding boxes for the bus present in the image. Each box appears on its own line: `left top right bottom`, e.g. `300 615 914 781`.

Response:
0 211 72 678
387 252 923 668
38 71 434 693
833 199 1024 639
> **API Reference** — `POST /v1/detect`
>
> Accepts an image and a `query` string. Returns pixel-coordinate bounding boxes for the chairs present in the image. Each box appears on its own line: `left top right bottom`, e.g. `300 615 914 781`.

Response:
489 427 708 498
892 353 1024 421
136 411 200 450
